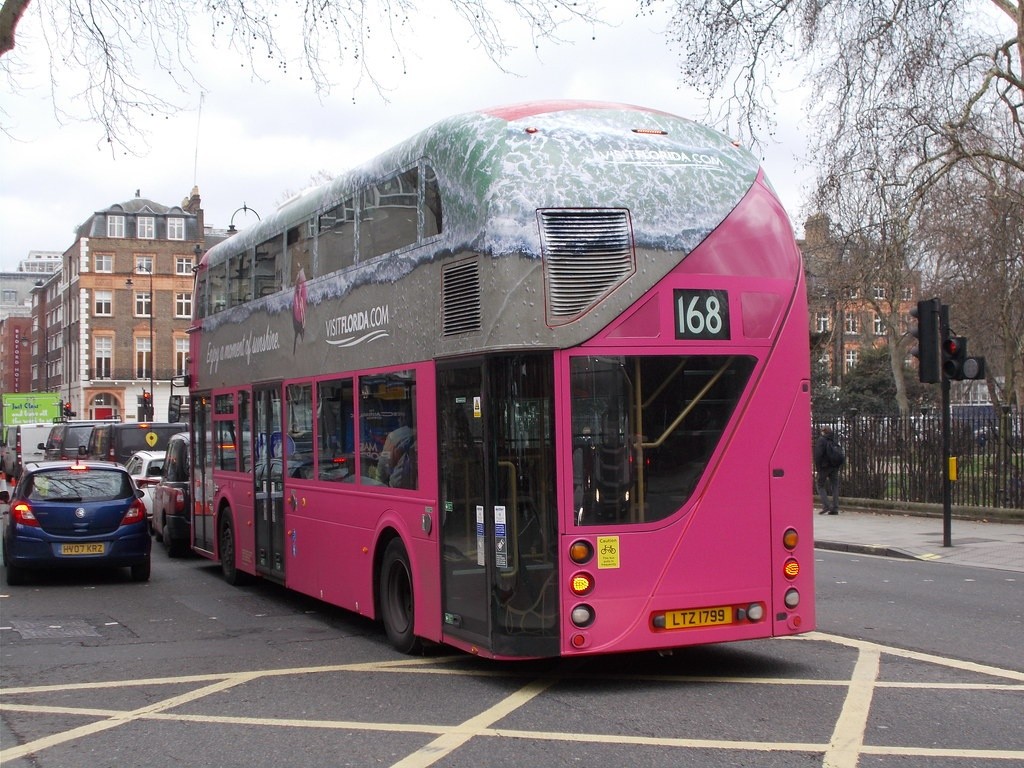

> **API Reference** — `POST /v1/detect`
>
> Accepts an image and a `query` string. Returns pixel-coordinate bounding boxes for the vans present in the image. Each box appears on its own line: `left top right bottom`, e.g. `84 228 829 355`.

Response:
148 430 257 557
37 422 188 468
0 423 61 479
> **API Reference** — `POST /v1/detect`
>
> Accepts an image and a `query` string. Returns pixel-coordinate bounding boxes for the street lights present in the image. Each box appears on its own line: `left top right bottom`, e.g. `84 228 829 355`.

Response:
125 265 154 420
20 324 49 393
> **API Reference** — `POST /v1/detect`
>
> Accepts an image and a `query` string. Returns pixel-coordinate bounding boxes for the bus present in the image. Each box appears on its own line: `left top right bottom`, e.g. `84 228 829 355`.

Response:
168 98 814 661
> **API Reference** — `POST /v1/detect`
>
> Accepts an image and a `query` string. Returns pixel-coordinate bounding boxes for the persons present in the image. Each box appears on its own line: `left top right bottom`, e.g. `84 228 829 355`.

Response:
377 391 444 485
815 425 839 515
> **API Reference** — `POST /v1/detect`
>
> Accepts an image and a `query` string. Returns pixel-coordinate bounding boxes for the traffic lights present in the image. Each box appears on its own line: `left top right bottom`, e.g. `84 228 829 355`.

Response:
143 393 151 415
909 299 938 385
940 335 965 380
64 403 70 415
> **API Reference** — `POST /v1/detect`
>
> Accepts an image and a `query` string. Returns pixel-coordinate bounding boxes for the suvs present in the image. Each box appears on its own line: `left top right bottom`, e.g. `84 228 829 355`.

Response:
1 459 153 586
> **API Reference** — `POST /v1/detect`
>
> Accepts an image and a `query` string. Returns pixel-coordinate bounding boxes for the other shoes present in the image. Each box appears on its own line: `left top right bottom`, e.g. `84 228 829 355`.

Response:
828 511 838 515
820 506 831 514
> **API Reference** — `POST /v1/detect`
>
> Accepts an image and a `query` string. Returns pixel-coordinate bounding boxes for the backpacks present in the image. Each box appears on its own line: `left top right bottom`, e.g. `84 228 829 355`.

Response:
824 437 843 466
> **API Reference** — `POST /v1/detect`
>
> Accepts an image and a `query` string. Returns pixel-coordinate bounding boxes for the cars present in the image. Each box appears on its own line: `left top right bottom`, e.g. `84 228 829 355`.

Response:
123 450 167 522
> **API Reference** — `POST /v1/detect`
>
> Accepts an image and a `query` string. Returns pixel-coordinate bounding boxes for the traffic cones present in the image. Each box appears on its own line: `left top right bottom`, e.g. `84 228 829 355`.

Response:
0 472 18 504
